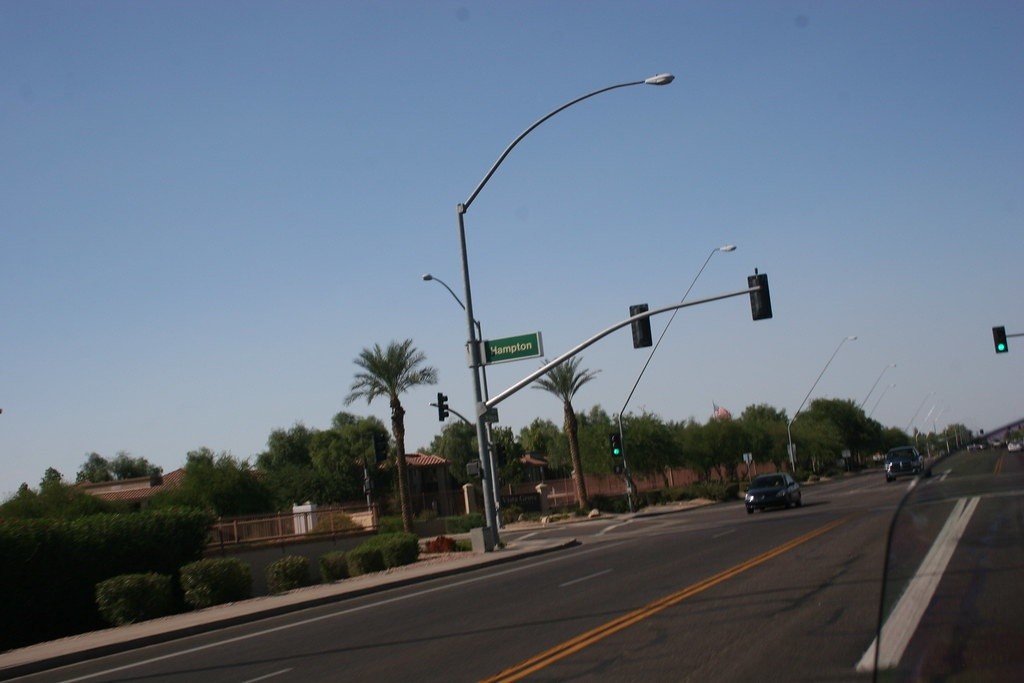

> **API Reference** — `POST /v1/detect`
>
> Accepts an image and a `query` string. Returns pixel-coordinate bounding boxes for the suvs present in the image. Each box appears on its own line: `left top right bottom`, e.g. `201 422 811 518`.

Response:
885 445 926 482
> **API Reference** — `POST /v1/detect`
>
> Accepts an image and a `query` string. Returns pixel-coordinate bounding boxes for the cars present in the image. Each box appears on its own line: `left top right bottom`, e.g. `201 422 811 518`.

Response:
744 473 800 514
966 437 1022 454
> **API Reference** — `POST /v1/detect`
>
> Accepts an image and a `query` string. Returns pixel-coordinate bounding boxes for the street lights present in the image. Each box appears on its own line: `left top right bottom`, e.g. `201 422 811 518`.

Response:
915 397 945 447
421 273 504 531
926 406 947 459
617 245 737 514
787 335 858 474
456 71 676 552
905 390 937 433
858 362 897 411
869 383 895 418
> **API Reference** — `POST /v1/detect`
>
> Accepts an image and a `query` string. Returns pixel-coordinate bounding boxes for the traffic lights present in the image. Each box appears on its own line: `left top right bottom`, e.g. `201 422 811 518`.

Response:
992 326 1009 354
609 432 622 457
438 392 449 421
